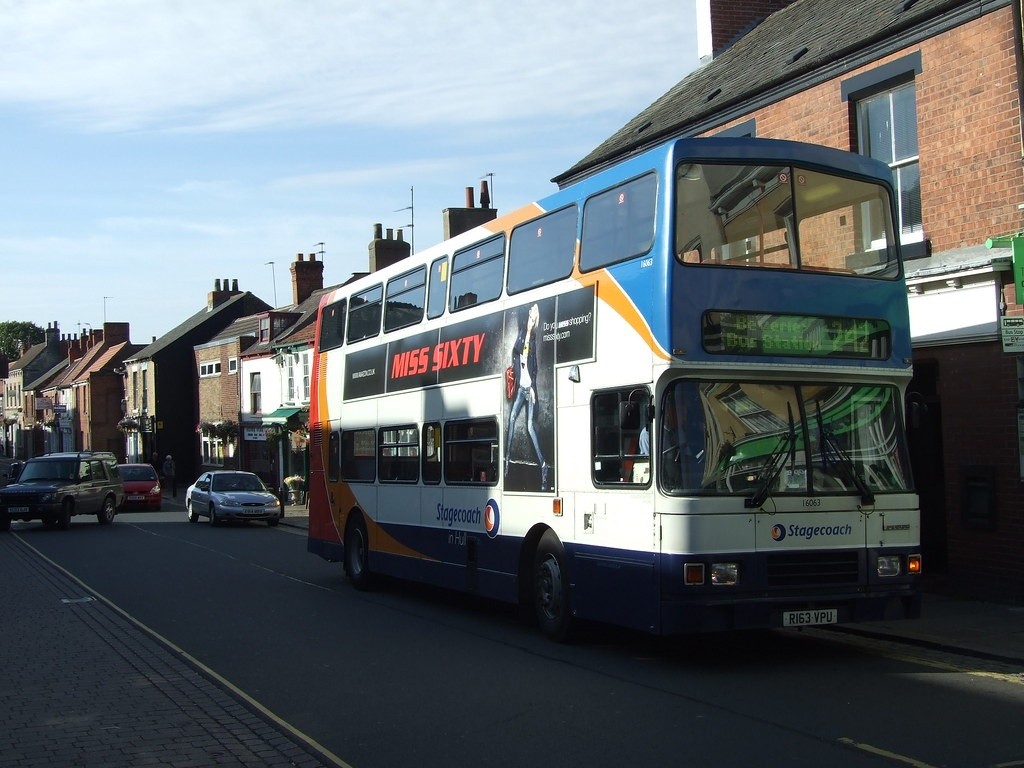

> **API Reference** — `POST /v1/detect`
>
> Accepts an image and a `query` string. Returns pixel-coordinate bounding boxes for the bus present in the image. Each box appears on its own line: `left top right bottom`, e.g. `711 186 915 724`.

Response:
306 139 923 640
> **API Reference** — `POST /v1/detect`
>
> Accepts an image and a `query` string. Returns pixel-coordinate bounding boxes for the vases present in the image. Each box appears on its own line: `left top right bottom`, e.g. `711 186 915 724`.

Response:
289 490 299 505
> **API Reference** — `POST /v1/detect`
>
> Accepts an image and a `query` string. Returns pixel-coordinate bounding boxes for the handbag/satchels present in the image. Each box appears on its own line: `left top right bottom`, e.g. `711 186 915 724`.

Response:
505 365 516 400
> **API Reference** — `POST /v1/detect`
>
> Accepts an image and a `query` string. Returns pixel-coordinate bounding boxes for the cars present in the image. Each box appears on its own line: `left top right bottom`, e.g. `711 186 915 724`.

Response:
3 462 25 485
186 470 281 528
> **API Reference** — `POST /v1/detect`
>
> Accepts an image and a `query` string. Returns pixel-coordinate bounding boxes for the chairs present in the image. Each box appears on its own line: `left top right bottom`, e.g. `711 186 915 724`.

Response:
213 480 227 491
389 460 473 481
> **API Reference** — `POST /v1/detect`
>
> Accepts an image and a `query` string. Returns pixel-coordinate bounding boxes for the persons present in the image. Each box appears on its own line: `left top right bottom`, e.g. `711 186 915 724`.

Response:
639 395 696 472
150 452 163 475
163 454 176 492
503 302 550 492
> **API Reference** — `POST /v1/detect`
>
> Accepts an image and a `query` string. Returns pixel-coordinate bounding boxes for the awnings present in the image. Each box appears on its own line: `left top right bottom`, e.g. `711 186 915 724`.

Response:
261 408 301 426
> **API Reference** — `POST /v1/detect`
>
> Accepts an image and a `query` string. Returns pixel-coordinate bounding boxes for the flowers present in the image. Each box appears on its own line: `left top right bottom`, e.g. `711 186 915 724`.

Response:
284 475 304 491
116 418 138 432
195 422 217 438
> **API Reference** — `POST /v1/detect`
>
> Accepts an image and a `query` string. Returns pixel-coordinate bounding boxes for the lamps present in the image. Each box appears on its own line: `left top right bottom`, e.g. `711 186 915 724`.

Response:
114 368 128 378
280 348 298 363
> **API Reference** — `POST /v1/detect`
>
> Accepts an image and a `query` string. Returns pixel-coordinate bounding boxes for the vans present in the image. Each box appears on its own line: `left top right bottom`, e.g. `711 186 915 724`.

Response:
118 464 161 512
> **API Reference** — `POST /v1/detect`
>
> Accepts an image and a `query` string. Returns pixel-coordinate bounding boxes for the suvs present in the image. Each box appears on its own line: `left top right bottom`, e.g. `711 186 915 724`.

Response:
0 451 126 531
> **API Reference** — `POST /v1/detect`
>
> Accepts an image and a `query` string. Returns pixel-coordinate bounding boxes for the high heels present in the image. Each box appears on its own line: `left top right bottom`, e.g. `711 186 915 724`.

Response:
541 466 549 491
504 452 510 477
